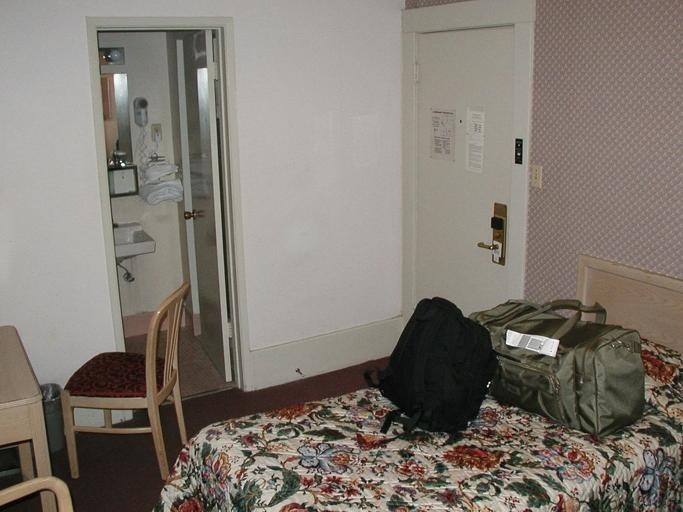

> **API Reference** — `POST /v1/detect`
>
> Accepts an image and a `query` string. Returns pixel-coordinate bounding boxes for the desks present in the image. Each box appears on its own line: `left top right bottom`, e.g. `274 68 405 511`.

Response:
0 325 57 512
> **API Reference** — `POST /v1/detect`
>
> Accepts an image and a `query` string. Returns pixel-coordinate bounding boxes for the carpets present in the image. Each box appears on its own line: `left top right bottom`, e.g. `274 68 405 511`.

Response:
126 328 233 405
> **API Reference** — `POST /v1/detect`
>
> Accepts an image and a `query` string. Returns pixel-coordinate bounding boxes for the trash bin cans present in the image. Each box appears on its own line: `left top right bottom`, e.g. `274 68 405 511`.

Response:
40 383 65 454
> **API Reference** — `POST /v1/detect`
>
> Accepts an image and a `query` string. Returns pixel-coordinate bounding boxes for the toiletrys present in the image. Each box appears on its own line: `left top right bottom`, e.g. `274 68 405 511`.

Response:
113 150 127 167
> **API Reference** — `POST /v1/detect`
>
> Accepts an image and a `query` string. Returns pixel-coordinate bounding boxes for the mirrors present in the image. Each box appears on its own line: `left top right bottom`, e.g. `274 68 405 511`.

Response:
102 73 133 165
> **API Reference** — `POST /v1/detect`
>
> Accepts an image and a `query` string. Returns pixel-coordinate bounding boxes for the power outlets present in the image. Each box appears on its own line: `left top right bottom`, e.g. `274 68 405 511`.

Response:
150 123 162 142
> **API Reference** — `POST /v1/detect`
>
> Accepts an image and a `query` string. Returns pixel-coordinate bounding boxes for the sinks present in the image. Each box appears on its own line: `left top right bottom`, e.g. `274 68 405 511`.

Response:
113 222 157 264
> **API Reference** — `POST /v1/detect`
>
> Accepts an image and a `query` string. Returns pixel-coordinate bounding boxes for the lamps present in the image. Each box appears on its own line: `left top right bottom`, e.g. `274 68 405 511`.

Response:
108 48 121 62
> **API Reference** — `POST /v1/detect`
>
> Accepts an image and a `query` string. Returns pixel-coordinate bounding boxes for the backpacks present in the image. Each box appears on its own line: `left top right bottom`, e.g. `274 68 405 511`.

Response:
363 297 493 434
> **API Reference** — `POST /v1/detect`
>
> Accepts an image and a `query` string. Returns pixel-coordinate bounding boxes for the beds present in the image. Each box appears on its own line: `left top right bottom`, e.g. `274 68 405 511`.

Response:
149 252 683 511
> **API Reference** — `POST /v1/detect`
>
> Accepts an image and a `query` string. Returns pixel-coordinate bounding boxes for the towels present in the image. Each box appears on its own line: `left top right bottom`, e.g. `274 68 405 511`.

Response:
141 178 183 205
143 165 179 181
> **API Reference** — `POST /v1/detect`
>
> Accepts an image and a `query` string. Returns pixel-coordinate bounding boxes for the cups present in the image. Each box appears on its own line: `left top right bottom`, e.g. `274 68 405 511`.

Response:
114 152 126 165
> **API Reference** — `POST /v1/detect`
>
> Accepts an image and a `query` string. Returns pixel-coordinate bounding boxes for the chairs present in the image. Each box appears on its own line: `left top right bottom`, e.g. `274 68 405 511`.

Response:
61 282 191 479
0 476 73 512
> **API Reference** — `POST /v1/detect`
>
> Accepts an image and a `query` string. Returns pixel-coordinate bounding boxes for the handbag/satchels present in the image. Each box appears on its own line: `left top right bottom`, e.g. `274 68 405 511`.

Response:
468 298 645 437
